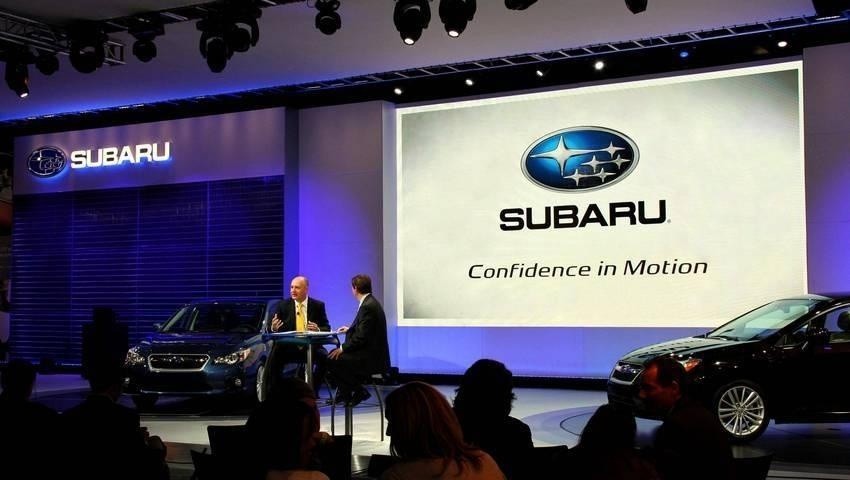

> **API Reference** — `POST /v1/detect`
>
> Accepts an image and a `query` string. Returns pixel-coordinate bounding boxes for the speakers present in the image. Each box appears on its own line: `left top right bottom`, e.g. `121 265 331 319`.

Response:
92 307 118 322
81 322 129 379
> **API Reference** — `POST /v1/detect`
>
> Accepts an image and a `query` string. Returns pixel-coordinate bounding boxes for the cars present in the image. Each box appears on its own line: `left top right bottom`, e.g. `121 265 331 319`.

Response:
125 296 317 412
605 291 849 446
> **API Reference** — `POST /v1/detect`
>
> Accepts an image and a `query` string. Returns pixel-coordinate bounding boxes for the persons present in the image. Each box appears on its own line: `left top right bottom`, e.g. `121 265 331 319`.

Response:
572 402 664 479
637 354 735 480
63 362 172 479
452 358 534 456
262 275 331 404
266 372 322 433
232 395 330 479
321 272 392 409
0 357 62 417
378 379 509 479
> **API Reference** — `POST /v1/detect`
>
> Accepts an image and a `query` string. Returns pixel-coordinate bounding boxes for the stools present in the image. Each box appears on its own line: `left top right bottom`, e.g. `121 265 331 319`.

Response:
322 367 399 441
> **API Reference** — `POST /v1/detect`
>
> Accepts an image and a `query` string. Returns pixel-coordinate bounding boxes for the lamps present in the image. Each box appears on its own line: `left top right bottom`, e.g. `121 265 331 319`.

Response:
392 2 432 46
314 0 343 35
594 61 613 73
535 65 554 79
439 2 477 39
394 81 411 99
464 73 482 87
5 1 261 100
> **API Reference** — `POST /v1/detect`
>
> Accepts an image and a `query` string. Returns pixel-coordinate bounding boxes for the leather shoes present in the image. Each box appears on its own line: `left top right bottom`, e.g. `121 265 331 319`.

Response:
326 388 372 407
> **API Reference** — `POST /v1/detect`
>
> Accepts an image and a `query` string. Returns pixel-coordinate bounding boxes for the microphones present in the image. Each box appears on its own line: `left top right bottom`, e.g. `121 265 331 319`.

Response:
297 312 299 315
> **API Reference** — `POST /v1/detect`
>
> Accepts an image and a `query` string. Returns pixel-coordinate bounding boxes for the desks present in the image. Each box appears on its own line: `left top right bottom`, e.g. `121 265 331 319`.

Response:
275 337 339 391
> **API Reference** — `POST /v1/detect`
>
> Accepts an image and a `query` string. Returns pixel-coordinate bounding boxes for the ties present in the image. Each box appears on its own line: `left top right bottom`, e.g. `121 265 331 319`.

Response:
296 304 308 349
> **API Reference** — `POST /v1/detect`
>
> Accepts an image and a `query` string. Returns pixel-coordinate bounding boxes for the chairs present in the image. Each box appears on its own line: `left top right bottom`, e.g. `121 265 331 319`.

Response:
829 310 850 343
